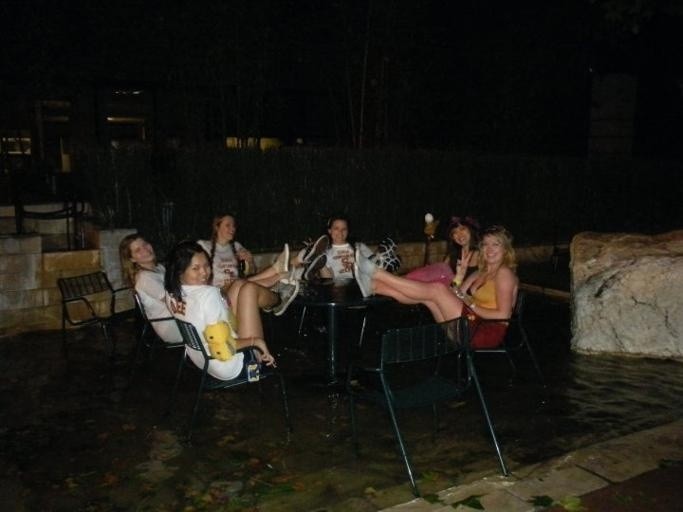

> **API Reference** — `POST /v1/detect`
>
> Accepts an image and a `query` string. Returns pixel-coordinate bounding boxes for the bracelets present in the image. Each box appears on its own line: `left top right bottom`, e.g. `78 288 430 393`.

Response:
251 335 256 346
470 302 476 309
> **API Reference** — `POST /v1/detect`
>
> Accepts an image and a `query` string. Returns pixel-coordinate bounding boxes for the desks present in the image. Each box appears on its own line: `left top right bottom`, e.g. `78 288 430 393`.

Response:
277 280 396 435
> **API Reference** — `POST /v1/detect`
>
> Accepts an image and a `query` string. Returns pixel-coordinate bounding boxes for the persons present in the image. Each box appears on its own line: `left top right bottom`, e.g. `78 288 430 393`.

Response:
355 212 480 300
317 214 403 279
353 224 520 350
119 233 296 345
193 212 328 287
163 240 300 382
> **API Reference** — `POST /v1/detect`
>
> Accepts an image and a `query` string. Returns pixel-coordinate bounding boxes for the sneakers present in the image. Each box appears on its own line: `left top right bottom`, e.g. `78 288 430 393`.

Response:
354 263 374 298
356 247 378 276
262 235 330 317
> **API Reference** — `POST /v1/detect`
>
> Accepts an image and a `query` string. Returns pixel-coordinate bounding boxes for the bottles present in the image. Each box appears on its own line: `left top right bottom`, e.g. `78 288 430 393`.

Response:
450 282 460 295
236 248 246 271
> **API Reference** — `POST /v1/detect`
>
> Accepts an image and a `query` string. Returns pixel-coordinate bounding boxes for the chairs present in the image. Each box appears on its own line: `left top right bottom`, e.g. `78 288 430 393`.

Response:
55 271 141 403
174 317 296 450
344 313 511 495
453 288 545 420
130 297 198 426
